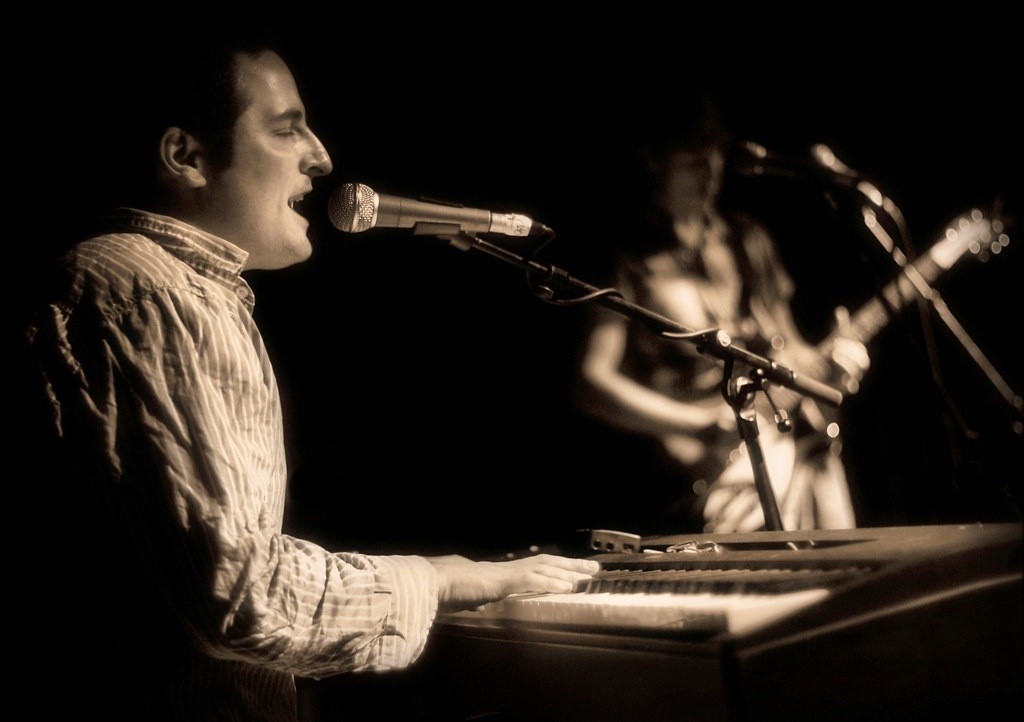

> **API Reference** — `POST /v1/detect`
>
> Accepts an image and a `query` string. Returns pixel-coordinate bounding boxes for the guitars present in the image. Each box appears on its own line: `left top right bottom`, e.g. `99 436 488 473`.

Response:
626 217 992 493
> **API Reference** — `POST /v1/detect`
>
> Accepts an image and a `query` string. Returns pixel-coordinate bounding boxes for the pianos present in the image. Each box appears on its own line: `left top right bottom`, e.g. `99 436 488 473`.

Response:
436 523 1024 722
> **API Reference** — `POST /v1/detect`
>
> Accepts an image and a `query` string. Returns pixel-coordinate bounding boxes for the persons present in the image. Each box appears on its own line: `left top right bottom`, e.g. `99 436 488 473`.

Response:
582 100 870 531
0 35 600 722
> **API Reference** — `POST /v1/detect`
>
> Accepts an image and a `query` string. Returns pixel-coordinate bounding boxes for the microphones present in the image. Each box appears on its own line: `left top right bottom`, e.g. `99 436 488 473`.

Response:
734 141 885 208
329 183 545 237
